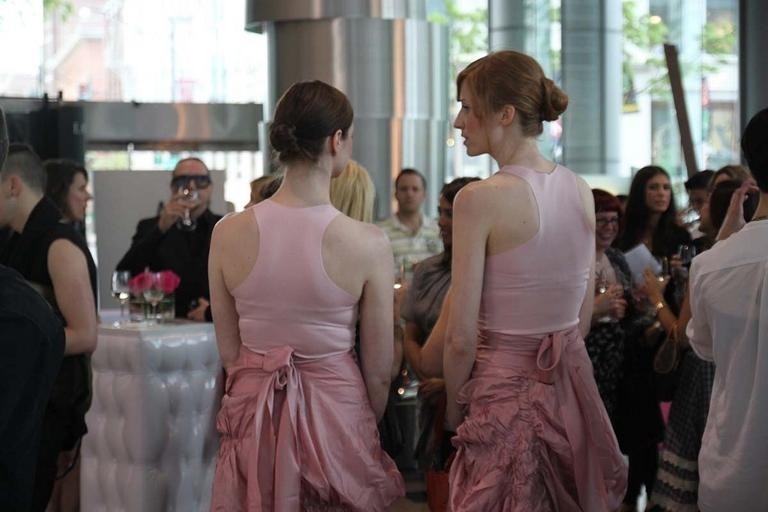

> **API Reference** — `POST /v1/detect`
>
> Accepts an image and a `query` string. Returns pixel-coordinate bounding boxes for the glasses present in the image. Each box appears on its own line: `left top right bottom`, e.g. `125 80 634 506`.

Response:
596 215 619 227
170 175 211 189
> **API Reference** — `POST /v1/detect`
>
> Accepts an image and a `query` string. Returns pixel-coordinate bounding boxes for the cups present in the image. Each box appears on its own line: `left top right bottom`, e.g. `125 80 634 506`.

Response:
649 254 668 280
674 205 701 235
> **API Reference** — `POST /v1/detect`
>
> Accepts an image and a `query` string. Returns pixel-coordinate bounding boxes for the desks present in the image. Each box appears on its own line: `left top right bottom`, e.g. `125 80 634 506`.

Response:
79 314 225 512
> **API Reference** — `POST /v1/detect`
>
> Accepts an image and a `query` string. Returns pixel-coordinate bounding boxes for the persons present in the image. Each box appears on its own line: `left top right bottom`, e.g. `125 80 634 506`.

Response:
0 141 98 512
118 158 224 320
245 107 766 510
446 49 630 510
41 159 91 512
0 105 65 512
208 78 407 512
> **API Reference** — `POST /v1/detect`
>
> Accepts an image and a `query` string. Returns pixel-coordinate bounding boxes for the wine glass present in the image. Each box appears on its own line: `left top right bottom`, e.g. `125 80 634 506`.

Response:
111 270 167 331
177 186 199 227
597 266 624 323
